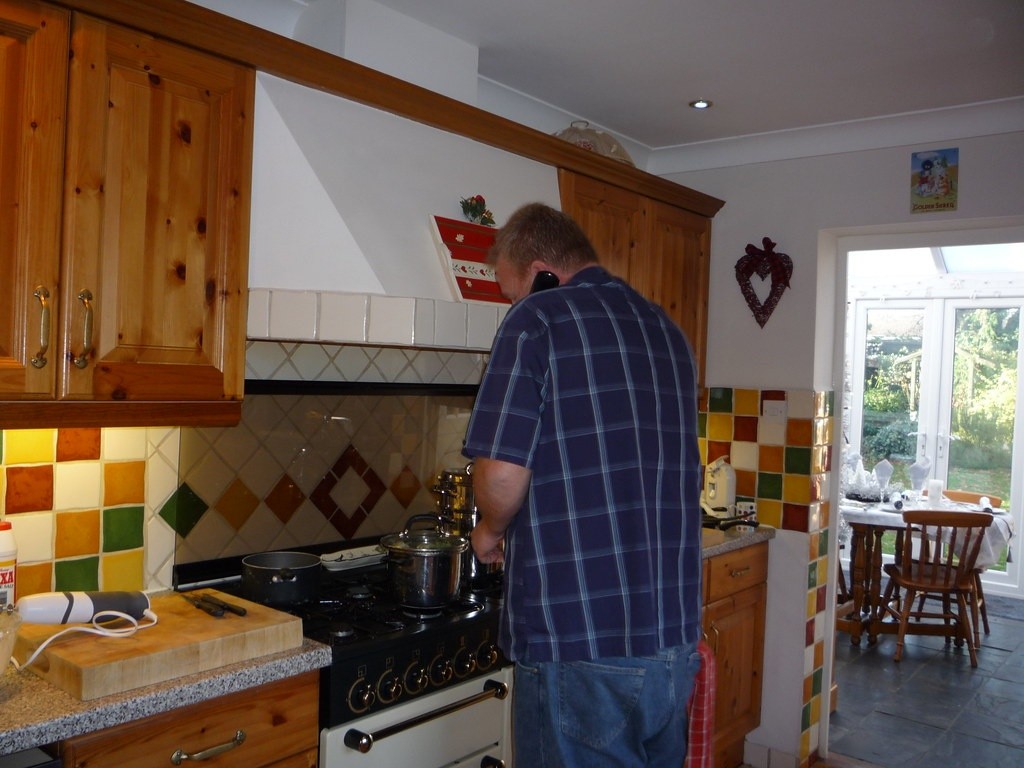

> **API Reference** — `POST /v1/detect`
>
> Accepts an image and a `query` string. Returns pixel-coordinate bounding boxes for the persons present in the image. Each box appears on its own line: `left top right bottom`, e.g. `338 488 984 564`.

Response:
462 202 702 768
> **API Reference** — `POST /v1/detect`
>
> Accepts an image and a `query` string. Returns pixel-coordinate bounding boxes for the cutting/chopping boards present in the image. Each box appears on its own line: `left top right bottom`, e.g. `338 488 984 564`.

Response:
9 587 304 699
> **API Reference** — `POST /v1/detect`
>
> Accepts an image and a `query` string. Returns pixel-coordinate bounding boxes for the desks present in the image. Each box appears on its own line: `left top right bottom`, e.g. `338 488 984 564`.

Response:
839 494 1016 645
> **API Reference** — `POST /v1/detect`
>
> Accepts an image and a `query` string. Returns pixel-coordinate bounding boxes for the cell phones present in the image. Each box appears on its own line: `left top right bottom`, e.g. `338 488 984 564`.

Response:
530 272 559 295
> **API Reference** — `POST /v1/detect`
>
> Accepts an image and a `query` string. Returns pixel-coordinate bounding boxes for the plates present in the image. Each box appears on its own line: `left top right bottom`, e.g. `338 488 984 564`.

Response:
970 506 1007 513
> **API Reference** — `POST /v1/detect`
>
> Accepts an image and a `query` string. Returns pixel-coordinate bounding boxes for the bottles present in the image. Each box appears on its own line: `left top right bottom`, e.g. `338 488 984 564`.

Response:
0 521 17 614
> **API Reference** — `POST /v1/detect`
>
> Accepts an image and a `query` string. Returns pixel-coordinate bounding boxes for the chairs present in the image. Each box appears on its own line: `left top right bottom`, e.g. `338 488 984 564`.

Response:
878 490 1001 669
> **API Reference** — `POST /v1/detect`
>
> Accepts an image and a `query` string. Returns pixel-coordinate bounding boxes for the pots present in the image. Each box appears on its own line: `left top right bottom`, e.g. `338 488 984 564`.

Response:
380 511 470 615
429 463 507 582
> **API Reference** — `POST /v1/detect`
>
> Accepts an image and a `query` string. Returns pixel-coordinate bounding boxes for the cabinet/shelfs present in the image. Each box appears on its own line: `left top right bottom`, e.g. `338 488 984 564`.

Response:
42 670 319 768
700 540 769 757
559 169 712 400
1 0 255 427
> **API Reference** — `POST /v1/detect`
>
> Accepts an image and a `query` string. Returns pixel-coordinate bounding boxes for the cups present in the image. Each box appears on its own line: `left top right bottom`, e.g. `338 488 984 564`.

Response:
728 501 758 533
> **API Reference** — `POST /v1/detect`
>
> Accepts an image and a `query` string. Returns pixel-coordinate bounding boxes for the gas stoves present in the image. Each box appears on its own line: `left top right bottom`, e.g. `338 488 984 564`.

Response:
173 531 518 728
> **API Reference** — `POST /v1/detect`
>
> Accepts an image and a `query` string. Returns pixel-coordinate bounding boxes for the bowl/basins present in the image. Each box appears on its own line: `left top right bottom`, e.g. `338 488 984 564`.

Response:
241 552 322 606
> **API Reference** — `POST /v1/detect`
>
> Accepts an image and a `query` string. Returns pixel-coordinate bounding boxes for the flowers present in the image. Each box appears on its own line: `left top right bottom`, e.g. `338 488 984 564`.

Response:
460 195 494 227
